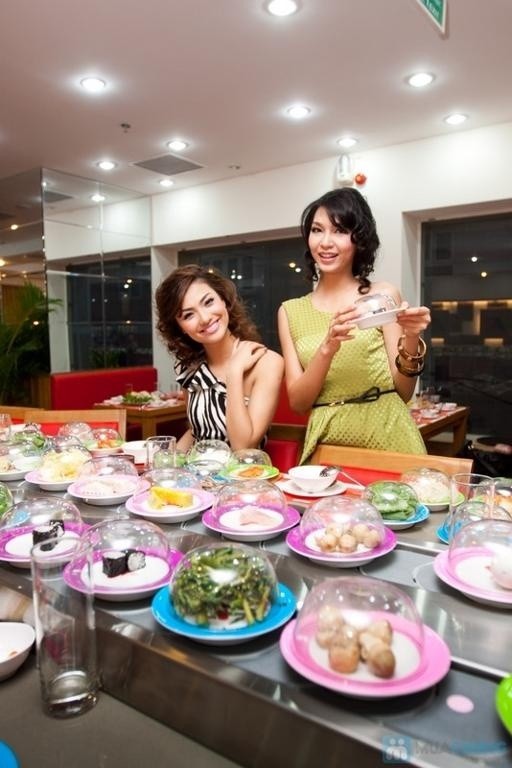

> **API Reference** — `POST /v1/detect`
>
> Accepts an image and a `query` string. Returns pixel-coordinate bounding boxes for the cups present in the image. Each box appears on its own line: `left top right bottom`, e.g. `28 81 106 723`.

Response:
29 536 101 719
146 435 177 469
448 473 495 542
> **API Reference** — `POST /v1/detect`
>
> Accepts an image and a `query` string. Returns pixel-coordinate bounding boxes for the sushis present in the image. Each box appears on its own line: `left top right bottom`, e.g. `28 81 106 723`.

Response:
101 551 126 578
124 549 147 572
33 518 65 551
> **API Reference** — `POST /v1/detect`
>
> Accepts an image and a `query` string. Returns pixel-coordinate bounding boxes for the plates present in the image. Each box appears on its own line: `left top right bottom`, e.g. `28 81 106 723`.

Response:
67 474 150 507
375 499 431 531
201 501 301 543
125 488 217 524
274 477 349 499
345 308 410 330
23 468 78 492
1 518 94 569
435 547 512 610
222 463 279 481
104 382 180 409
62 547 185 601
416 488 466 512
122 439 158 466
156 452 236 471
285 517 399 567
409 391 461 418
278 610 452 698
435 519 466 546
1 455 43 481
151 579 299 644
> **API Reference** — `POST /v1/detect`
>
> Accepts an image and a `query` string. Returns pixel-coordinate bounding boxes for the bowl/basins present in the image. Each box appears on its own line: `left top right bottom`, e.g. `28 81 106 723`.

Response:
287 464 339 490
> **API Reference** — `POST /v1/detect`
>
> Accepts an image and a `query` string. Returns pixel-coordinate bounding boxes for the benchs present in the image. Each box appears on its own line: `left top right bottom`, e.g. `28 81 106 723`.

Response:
53 367 158 411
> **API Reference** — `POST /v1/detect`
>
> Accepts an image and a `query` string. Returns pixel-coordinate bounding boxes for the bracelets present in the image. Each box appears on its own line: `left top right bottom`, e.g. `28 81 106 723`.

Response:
397 335 427 361
395 354 425 378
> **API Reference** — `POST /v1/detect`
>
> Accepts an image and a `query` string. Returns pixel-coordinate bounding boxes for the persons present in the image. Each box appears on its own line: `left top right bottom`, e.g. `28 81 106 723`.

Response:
277 186 432 465
154 263 285 454
